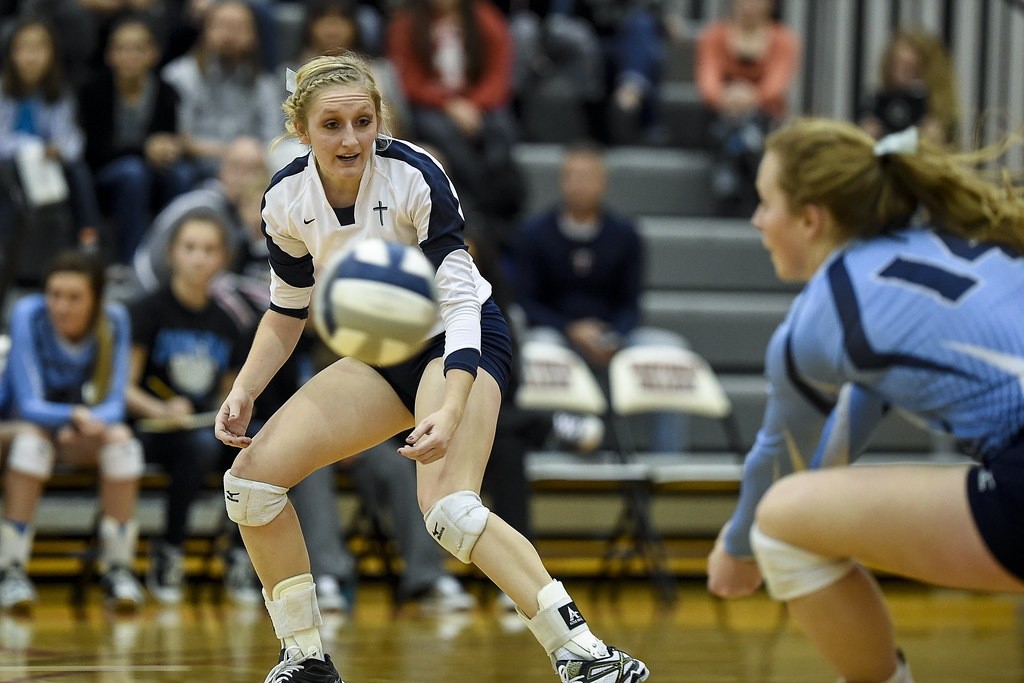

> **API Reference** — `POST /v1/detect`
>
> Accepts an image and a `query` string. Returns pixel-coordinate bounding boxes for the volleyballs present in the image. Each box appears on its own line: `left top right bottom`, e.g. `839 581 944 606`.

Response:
312 236 440 368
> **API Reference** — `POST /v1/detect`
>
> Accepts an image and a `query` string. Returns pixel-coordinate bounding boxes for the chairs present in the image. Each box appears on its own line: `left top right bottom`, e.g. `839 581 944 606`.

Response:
42 327 755 606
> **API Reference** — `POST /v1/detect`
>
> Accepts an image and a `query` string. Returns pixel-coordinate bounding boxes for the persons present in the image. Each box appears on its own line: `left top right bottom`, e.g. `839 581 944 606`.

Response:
213 58 649 683
707 119 1023 683
0 0 964 608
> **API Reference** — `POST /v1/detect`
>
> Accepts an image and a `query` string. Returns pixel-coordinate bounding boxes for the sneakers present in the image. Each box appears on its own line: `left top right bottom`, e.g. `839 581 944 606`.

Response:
262 645 344 683
554 646 650 683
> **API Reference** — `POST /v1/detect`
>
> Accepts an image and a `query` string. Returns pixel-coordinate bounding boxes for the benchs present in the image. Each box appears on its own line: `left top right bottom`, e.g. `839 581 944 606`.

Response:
31 76 806 540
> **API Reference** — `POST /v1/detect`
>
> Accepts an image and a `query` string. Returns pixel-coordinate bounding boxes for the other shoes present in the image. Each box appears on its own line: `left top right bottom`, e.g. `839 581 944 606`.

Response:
315 574 349 615
498 590 515 612
0 565 37 611
220 549 264 607
100 563 149 611
546 408 605 454
417 574 480 615
147 541 185 606
